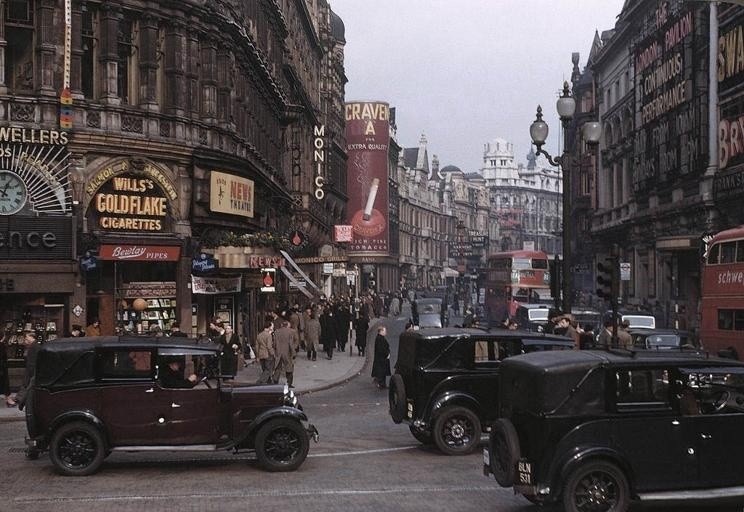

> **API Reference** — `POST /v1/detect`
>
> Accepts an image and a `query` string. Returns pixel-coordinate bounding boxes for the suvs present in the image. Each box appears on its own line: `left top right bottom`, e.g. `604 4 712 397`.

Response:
385 326 577 456
515 305 550 331
482 340 743 512
629 329 697 351
411 297 443 329
600 310 656 329
18 336 320 479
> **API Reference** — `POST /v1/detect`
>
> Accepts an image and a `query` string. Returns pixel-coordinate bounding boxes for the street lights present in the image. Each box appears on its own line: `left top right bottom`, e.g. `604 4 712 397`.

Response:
528 81 603 314
116 296 148 336
454 218 468 295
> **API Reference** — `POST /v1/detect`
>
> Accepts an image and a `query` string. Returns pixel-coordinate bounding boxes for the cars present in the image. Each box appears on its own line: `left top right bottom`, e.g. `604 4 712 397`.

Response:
432 285 453 301
477 287 485 306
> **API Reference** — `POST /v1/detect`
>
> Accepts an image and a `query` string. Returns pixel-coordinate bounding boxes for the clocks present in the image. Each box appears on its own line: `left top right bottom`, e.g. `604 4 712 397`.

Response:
0 169 28 216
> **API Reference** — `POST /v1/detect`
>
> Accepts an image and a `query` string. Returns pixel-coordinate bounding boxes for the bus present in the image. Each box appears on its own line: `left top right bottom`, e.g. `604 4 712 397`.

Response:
485 249 552 324
694 225 744 361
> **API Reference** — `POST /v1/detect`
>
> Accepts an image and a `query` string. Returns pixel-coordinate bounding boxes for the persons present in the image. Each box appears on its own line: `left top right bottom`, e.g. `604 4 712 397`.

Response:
86 317 102 337
439 278 690 352
70 325 85 337
158 316 241 390
0 332 16 408
13 332 40 404
255 284 419 389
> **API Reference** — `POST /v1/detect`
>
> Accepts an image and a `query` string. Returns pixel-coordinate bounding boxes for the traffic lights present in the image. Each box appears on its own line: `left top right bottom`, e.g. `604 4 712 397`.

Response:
595 259 620 305
